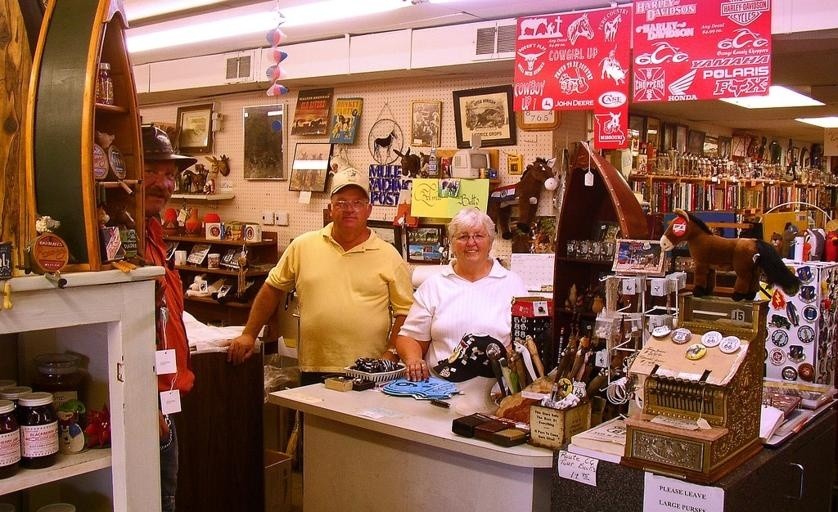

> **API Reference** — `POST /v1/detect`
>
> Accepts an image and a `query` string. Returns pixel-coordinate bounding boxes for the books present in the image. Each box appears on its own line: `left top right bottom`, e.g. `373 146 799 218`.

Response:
629 177 834 213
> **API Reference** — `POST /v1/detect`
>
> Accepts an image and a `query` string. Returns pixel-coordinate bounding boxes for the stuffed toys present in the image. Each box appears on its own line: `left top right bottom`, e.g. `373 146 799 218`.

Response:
655 205 803 303
483 154 563 241
768 220 837 264
393 145 420 176
416 152 431 174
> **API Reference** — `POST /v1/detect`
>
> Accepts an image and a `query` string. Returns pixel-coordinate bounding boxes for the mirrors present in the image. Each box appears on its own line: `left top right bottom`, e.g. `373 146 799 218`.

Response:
241 103 288 181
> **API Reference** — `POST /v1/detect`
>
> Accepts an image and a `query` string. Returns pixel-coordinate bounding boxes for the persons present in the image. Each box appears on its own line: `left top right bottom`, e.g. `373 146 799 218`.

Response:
216 166 415 388
137 120 200 512
391 206 538 385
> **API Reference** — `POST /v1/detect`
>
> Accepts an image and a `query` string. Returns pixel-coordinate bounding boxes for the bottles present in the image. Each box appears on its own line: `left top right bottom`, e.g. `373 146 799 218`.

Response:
184 208 202 238
427 148 450 179
96 61 115 106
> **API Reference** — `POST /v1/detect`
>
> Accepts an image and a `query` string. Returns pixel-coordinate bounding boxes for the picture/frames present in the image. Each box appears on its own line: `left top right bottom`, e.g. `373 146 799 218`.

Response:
408 98 442 148
288 141 332 194
174 102 214 154
629 113 810 167
451 84 516 149
406 223 447 264
366 219 403 261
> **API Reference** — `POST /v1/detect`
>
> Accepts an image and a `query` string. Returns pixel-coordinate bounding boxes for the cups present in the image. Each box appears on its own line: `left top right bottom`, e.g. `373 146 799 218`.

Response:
174 250 186 266
207 253 220 270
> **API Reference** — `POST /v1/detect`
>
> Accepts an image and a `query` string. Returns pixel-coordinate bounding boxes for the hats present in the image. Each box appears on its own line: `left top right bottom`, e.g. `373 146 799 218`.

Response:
434 333 507 382
141 127 197 175
330 168 369 202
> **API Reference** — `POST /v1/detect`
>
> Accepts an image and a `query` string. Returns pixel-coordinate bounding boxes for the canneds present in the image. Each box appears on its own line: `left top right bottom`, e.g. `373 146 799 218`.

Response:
0 379 59 479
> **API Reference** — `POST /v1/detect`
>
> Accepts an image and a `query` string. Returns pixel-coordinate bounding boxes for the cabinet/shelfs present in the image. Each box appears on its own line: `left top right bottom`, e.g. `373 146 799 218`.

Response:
629 174 838 226
167 221 278 346
0 0 167 512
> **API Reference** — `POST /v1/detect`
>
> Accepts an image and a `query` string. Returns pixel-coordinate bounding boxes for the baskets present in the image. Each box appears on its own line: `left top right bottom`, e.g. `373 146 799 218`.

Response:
344 360 407 383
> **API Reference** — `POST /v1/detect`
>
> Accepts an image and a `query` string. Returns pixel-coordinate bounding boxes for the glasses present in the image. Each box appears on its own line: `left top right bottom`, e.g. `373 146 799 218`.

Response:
333 200 366 209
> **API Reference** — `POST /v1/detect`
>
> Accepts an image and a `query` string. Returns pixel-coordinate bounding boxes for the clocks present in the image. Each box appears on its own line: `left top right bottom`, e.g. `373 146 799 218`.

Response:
517 110 562 132
